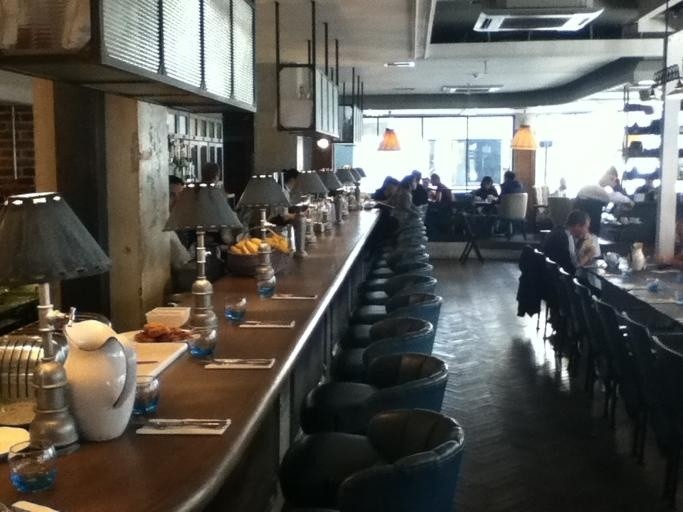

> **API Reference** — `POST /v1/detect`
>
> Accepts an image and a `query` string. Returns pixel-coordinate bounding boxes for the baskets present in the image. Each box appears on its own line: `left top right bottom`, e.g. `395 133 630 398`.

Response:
227 227 293 278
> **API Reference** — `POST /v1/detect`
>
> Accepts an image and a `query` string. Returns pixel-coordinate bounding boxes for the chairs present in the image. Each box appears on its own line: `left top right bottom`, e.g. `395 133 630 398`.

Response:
548 197 572 229
533 205 553 239
329 318 434 382
371 245 428 278
407 218 427 237
638 335 683 510
301 352 449 435
348 292 443 346
537 257 558 340
551 267 570 356
493 193 528 240
373 236 427 269
586 295 617 420
354 274 437 323
524 245 544 272
560 278 591 377
281 409 464 512
573 196 607 237
363 254 431 290
361 263 433 305
610 312 650 455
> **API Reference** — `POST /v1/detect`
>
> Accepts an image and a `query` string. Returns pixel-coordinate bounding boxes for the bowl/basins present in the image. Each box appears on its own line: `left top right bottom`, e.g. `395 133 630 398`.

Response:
147 306 192 329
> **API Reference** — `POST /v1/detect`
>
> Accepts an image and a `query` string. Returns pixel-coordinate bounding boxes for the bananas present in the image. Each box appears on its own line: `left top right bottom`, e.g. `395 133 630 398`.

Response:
229 237 261 254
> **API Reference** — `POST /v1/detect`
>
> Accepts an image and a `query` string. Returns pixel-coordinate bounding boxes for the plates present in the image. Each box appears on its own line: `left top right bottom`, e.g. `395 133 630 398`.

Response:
0 426 29 457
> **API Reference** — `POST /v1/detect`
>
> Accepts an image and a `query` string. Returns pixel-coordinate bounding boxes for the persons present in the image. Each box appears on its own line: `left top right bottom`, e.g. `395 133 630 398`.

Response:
426 173 453 241
575 167 655 209
265 168 310 230
169 176 215 262
470 176 500 200
541 208 597 278
498 170 523 240
371 170 431 217
168 231 197 275
654 203 682 270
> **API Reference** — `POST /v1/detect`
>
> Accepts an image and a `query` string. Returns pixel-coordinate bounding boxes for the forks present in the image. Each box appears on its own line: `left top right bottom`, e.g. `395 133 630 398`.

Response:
199 357 271 365
128 417 227 426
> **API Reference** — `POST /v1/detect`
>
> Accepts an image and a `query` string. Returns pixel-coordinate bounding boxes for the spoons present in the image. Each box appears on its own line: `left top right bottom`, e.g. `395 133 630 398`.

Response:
151 422 224 430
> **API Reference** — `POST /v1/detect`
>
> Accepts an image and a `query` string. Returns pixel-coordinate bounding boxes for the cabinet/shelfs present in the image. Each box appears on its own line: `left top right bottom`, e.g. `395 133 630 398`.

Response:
0 0 257 114
275 0 338 142
331 68 363 146
169 109 224 187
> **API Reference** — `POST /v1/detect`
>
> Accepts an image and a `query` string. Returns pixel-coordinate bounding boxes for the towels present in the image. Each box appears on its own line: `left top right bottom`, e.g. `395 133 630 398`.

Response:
605 274 621 276
10 501 57 512
272 294 317 299
240 321 295 327
618 284 646 289
205 358 275 369
583 265 603 268
650 269 679 274
646 298 675 304
136 419 232 435
676 318 683 324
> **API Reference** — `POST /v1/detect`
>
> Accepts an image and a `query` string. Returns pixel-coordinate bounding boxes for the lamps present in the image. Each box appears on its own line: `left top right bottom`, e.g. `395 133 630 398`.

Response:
510 110 538 150
0 192 112 450
234 175 293 288
290 171 330 241
335 168 366 209
378 111 400 150
161 184 243 334
313 138 328 150
317 169 343 230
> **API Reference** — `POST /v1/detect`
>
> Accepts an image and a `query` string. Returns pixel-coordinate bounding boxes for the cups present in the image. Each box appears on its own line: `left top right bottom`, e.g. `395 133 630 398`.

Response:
255 272 277 298
133 375 159 416
187 327 216 359
7 438 58 493
223 295 246 322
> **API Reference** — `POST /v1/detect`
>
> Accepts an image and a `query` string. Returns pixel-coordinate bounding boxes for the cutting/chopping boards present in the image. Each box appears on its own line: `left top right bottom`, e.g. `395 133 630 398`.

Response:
117 328 194 386
